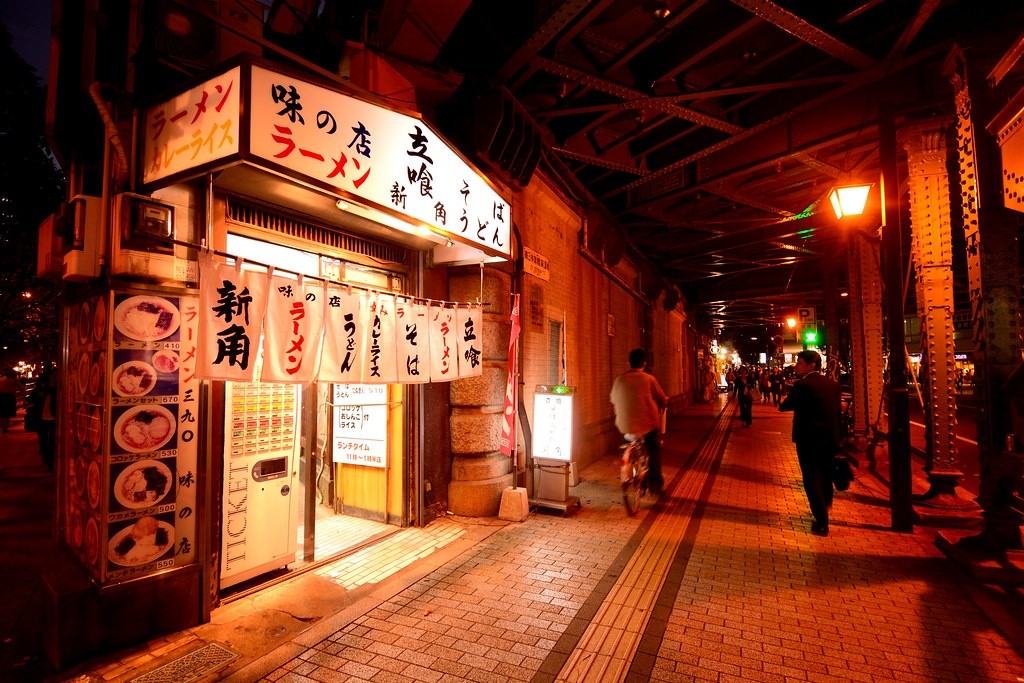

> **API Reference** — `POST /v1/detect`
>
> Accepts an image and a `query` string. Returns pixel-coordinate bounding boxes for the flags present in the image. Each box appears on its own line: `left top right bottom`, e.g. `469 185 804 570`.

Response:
499 295 519 456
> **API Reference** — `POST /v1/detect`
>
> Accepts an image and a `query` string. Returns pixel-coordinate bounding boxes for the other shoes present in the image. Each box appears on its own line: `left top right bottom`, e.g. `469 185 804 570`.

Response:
809 516 830 538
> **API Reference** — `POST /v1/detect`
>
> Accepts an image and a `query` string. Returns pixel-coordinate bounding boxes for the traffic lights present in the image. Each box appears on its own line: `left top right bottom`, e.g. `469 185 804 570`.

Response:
804 331 817 343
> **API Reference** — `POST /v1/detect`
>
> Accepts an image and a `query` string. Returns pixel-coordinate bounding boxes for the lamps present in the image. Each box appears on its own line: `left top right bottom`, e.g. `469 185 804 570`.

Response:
826 155 875 221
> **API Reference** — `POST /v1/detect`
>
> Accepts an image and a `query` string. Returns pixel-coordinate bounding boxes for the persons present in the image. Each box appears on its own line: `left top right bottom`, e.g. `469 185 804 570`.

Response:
735 366 753 425
701 356 782 405
778 350 845 538
611 347 670 492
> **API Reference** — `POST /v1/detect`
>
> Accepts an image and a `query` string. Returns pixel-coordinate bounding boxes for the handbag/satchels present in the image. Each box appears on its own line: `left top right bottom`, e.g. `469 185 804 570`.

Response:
23 406 43 432
834 452 855 492
748 386 764 402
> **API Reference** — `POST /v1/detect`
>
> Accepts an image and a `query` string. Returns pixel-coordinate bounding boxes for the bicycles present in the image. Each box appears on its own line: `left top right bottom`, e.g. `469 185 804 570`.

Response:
620 396 669 517
